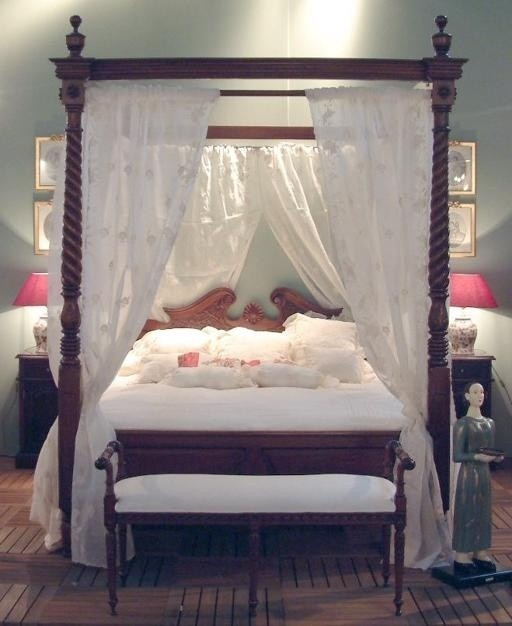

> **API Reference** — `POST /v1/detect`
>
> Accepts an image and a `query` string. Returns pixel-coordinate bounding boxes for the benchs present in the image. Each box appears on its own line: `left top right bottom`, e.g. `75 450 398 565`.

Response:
96 432 416 618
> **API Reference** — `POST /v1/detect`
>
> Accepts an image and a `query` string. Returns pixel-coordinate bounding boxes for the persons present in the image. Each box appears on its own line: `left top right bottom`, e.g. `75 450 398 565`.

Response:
450 378 506 570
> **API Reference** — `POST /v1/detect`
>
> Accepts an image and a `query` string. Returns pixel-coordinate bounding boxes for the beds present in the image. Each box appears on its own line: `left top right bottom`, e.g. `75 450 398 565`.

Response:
28 11 467 560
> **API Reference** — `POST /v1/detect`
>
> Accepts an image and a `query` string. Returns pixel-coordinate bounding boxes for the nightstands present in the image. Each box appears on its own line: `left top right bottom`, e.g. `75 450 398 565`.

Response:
450 347 494 420
13 349 60 468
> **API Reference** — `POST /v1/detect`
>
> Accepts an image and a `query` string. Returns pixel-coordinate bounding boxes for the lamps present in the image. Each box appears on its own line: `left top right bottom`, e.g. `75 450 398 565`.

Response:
12 273 63 354
450 272 496 356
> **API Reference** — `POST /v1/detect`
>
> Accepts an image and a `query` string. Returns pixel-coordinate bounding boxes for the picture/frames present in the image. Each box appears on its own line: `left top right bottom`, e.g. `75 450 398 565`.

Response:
34 136 63 190
34 199 54 256
448 202 476 259
446 140 476 195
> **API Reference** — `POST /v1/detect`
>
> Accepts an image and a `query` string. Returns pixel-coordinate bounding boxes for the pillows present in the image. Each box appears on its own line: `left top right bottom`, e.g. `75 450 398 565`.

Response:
127 313 362 389
